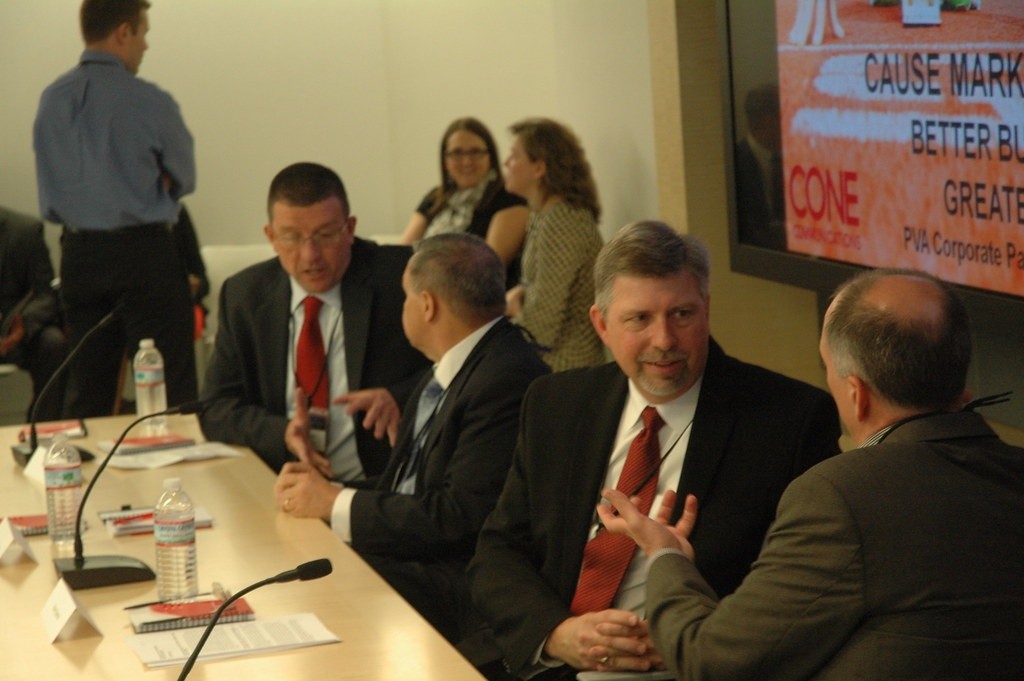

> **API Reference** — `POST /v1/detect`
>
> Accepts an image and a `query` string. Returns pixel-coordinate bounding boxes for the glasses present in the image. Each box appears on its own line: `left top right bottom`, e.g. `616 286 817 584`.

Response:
445 147 490 159
274 219 349 252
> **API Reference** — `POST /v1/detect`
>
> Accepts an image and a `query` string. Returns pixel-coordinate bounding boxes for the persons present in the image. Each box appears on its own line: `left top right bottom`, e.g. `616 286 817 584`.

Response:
32 0 197 422
0 204 69 423
596 267 1024 680
441 219 843 681
199 161 434 482
401 116 529 293
272 233 553 648
490 118 611 370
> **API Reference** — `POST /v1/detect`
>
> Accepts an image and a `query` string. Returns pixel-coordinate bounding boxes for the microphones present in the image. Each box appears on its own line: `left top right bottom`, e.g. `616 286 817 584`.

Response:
177 559 333 681
11 301 132 467
53 400 206 592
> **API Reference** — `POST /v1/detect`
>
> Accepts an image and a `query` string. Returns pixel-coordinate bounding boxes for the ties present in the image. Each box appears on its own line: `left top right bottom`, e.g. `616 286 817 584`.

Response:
569 405 665 616
295 294 328 453
395 374 442 493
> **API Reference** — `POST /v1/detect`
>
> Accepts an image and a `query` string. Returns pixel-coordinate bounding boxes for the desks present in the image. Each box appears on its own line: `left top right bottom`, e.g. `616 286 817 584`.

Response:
1 412 487 681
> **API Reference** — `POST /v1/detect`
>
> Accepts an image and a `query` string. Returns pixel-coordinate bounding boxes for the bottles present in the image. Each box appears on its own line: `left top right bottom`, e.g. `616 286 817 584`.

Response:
153 477 199 601
132 338 167 430
43 432 84 543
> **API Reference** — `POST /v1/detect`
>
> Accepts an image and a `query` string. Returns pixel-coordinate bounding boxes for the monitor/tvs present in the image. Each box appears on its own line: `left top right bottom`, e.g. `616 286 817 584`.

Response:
720 0 1024 335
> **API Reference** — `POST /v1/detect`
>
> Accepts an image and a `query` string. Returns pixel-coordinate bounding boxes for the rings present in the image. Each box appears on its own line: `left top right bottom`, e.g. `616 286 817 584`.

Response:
283 499 290 513
600 656 612 666
